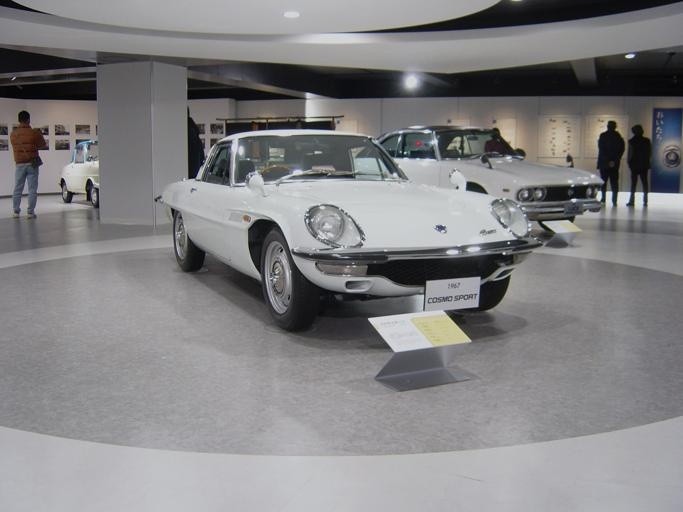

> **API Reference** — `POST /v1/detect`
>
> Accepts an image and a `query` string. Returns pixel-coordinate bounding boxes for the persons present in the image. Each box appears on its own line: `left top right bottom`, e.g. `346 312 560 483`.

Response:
188 106 205 179
10 110 46 220
625 123 652 207
596 121 625 208
484 127 506 155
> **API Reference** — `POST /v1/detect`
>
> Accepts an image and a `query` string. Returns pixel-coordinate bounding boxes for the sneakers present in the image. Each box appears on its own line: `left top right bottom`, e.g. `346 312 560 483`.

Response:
12 211 20 219
27 212 38 220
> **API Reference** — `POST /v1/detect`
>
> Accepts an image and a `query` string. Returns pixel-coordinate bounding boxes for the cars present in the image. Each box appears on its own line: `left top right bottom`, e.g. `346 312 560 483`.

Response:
59 134 98 206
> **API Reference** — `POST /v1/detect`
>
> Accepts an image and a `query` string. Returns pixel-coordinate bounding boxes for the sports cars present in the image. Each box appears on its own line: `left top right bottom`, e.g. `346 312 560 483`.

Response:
153 128 536 336
355 124 607 226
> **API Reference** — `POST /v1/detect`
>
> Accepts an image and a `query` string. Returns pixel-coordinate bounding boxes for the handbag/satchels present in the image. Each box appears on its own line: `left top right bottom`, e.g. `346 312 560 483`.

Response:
29 156 43 168
